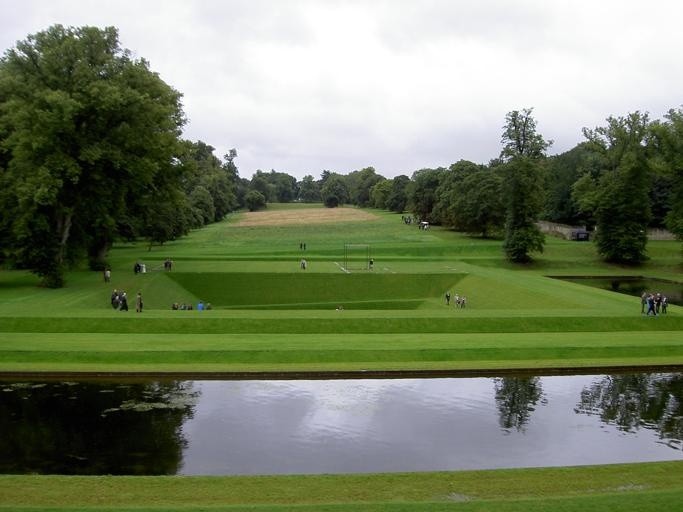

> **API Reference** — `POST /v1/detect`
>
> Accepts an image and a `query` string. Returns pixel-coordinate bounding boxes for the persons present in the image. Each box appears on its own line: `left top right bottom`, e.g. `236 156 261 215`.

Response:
400 216 428 230
640 292 668 316
105 269 111 283
133 262 140 273
135 293 142 312
111 288 128 312
164 262 171 268
454 293 459 306
460 294 466 308
197 300 203 309
369 258 373 267
206 302 211 310
172 303 192 310
140 264 145 272
302 259 306 269
445 291 450 305
